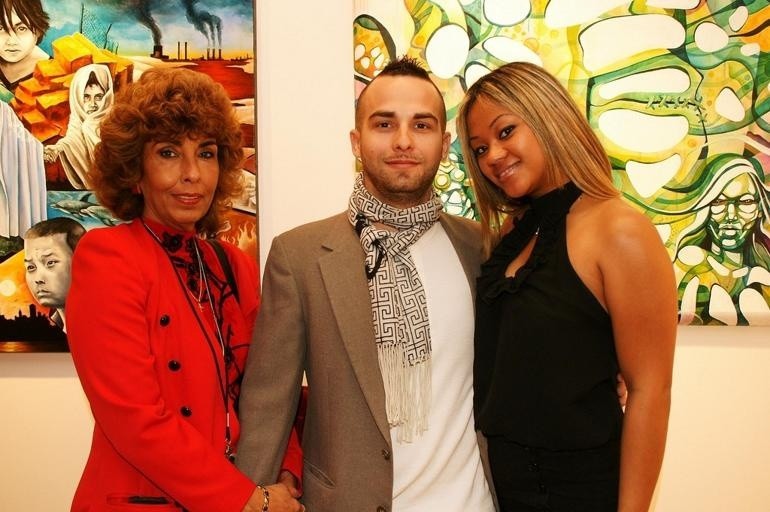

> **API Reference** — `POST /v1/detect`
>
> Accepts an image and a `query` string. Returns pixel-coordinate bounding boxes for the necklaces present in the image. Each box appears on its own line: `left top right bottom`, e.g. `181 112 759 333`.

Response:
142 222 205 316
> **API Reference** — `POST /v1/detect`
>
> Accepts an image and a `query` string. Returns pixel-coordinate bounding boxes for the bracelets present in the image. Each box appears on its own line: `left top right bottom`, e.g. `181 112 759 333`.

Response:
255 483 271 512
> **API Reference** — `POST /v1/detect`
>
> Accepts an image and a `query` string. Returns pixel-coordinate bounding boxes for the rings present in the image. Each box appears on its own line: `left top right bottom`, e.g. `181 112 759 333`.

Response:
301 504 307 512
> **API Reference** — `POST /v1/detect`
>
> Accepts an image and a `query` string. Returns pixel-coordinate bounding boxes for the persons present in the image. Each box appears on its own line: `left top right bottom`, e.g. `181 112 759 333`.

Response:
231 54 631 512
0 1 51 106
62 63 303 511
23 216 86 336
455 61 681 512
48 64 116 191
663 151 770 325
